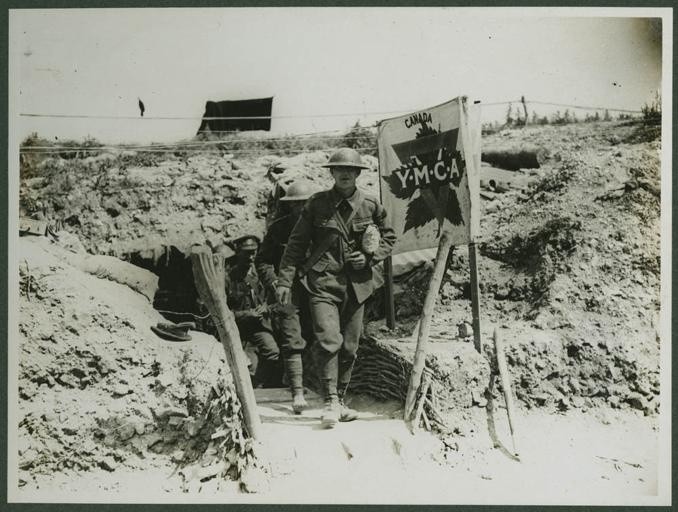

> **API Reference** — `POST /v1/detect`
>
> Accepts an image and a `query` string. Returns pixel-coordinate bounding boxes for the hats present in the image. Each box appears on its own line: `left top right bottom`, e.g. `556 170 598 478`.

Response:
232 234 261 251
279 181 312 201
150 321 196 341
320 147 371 170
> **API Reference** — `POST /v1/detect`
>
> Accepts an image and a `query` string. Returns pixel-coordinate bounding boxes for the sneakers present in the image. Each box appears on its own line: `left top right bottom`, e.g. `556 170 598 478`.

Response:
292 394 308 414
338 403 359 423
320 407 338 427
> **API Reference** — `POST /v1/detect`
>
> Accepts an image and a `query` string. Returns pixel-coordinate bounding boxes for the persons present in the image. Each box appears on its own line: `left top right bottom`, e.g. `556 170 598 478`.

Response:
254 181 314 415
220 234 280 392
272 147 397 429
